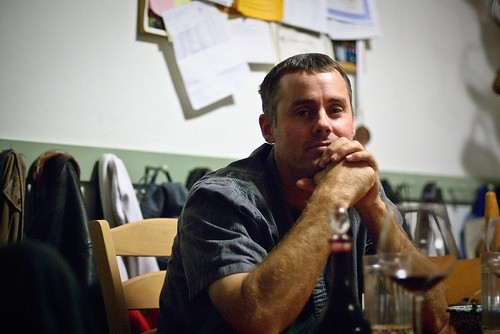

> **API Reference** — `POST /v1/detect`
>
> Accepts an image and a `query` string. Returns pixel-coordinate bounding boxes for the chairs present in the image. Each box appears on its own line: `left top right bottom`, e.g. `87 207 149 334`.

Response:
88 218 178 334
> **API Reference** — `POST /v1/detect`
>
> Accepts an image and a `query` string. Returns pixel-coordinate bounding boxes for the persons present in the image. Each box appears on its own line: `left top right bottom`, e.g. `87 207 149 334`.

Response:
156 53 451 334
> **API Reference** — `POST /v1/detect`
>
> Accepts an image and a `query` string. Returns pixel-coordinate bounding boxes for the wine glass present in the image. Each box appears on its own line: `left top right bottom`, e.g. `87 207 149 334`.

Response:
376 201 457 334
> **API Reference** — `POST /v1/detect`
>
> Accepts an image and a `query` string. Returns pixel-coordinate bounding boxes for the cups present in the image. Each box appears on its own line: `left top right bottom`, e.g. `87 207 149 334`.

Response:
362 250 415 334
446 304 483 334
479 251 500 334
